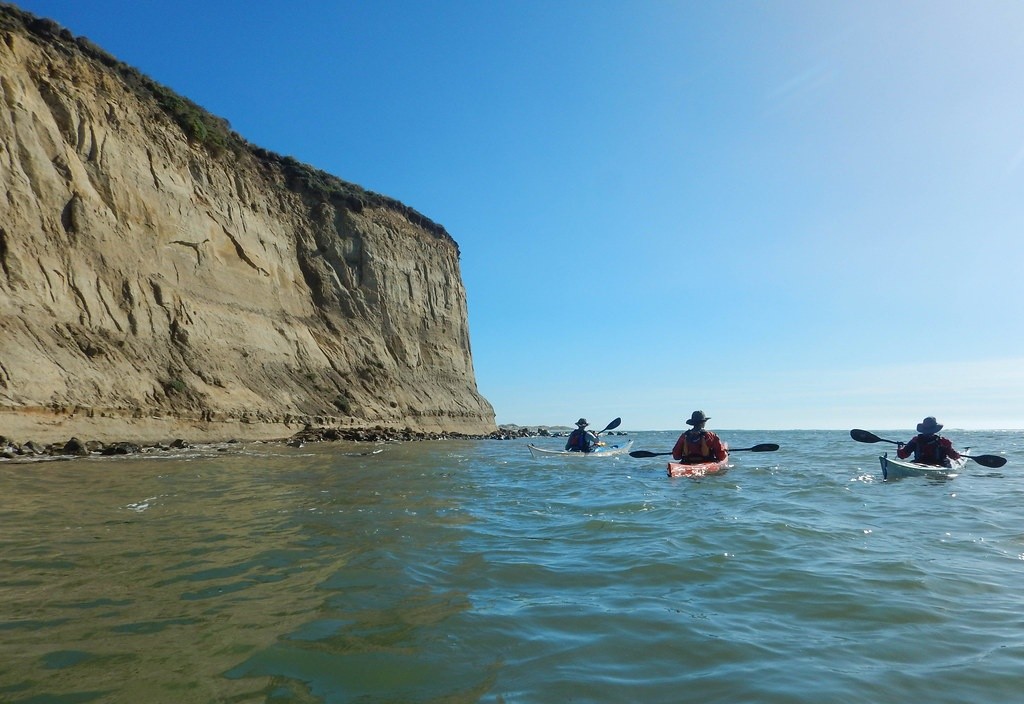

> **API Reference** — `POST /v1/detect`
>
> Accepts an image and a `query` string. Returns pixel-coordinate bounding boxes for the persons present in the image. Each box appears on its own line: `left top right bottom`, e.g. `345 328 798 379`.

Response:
672 410 728 464
897 416 960 468
565 418 599 452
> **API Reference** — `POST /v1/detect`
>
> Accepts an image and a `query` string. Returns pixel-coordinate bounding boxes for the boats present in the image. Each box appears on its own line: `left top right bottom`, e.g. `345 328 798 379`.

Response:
878 448 971 483
526 439 634 460
667 441 729 479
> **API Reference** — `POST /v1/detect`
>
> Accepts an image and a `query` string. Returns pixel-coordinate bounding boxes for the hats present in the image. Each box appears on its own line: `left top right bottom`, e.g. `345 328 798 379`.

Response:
575 418 590 427
686 411 711 425
917 417 943 434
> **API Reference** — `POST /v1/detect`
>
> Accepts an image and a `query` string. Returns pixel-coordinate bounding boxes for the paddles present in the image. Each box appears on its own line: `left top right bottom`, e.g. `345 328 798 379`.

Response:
569 416 622 452
849 428 1008 469
628 443 780 458
